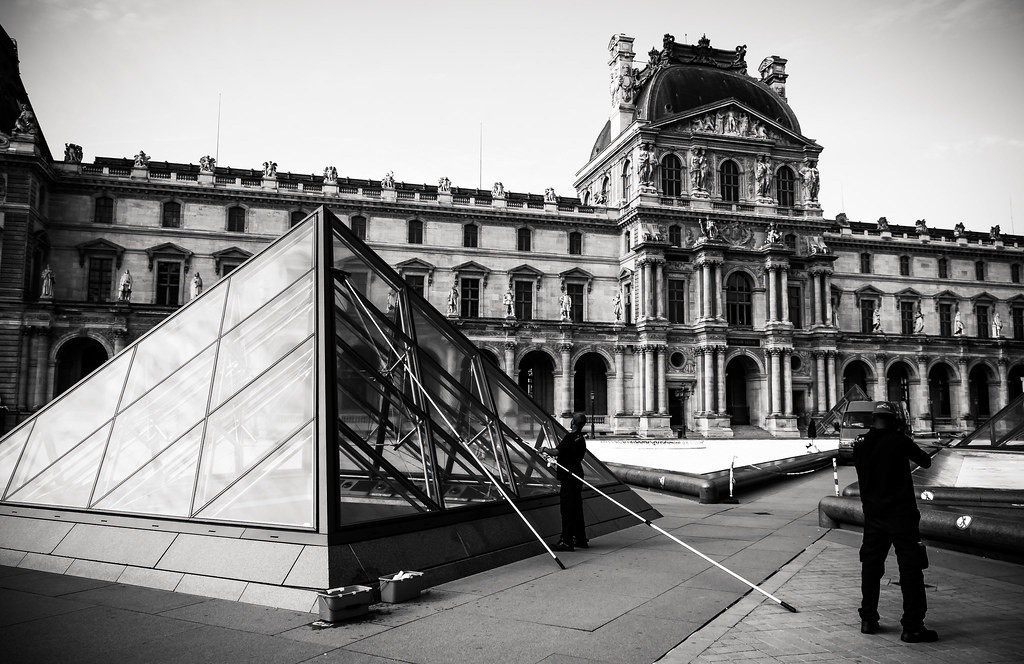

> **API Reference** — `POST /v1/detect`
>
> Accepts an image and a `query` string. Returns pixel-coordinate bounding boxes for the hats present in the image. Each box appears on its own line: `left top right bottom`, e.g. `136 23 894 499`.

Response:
872 401 899 416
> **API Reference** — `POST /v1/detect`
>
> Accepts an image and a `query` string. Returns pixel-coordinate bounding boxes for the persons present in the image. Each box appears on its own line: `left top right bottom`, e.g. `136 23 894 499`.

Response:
954 311 964 334
118 269 132 300
189 271 203 300
39 264 57 298
538 413 588 552
447 284 459 315
503 288 515 316
915 308 924 332
637 143 820 204
853 401 939 643
873 308 881 330
386 286 399 312
559 291 572 319
993 312 1002 336
613 293 623 321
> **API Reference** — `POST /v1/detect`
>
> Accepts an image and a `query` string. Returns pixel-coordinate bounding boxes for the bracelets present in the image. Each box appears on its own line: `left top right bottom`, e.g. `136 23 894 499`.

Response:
543 447 546 452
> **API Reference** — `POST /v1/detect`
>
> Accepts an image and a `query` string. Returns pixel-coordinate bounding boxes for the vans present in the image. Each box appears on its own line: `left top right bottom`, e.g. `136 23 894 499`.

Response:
834 398 915 466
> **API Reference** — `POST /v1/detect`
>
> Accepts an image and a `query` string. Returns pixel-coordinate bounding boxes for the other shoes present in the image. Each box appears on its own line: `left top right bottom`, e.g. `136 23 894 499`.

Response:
861 620 880 634
549 536 574 551
573 536 589 548
900 627 938 643
